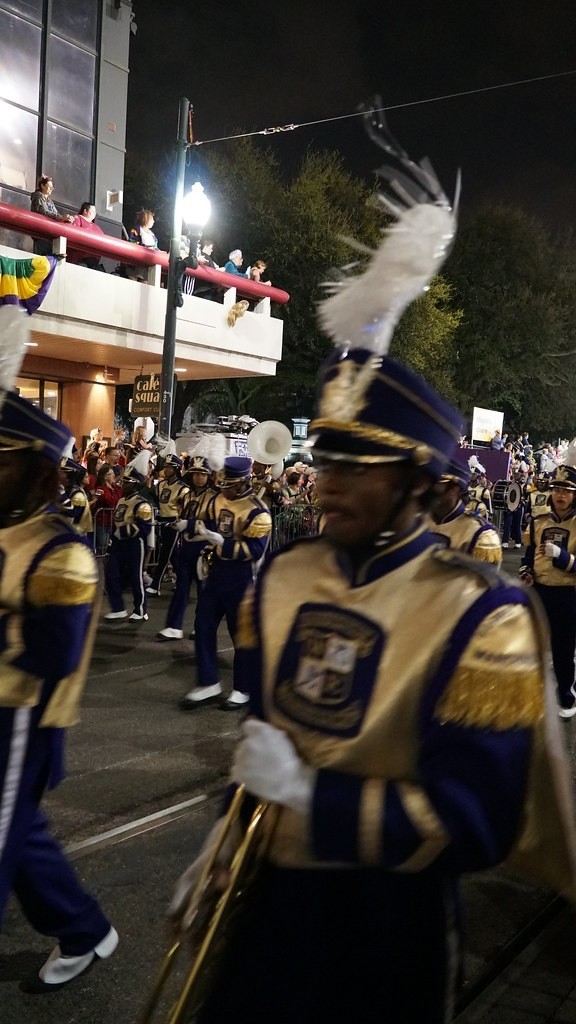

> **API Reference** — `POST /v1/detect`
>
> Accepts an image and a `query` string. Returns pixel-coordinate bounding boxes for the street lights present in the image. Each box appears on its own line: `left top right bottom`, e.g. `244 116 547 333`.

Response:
157 180 213 438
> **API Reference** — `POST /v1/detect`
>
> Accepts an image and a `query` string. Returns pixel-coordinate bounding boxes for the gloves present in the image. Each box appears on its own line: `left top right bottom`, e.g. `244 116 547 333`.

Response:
202 528 224 546
230 719 315 816
173 520 188 531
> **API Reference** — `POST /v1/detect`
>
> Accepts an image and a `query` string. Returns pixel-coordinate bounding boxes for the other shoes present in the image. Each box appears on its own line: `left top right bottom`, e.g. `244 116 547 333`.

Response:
220 699 252 711
38 925 119 983
501 542 508 550
129 612 149 622
155 628 184 641
514 543 521 549
178 691 223 709
188 631 197 640
103 609 128 619
557 702 576 722
145 587 161 595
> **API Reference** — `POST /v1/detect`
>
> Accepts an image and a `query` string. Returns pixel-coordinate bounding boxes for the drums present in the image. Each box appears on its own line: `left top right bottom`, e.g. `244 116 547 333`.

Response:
491 479 522 513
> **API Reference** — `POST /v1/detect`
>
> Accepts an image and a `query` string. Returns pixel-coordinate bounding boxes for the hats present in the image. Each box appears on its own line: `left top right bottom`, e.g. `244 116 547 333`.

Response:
162 438 183 470
187 437 212 475
0 305 73 460
305 157 467 476
438 456 473 490
547 436 576 493
57 436 87 478
207 434 251 489
536 460 553 482
467 455 486 478
120 450 153 485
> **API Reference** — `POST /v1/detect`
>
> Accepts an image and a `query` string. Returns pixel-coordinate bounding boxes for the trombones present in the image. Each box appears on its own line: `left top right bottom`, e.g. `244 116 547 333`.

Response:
138 783 275 1024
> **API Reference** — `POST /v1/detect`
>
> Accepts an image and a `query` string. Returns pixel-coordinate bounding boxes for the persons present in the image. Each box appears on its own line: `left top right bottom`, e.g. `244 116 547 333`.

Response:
273 458 328 549
121 206 167 284
67 201 106 268
168 221 271 312
30 175 75 256
168 348 576 1024
414 429 576 725
68 426 272 710
0 385 120 983
